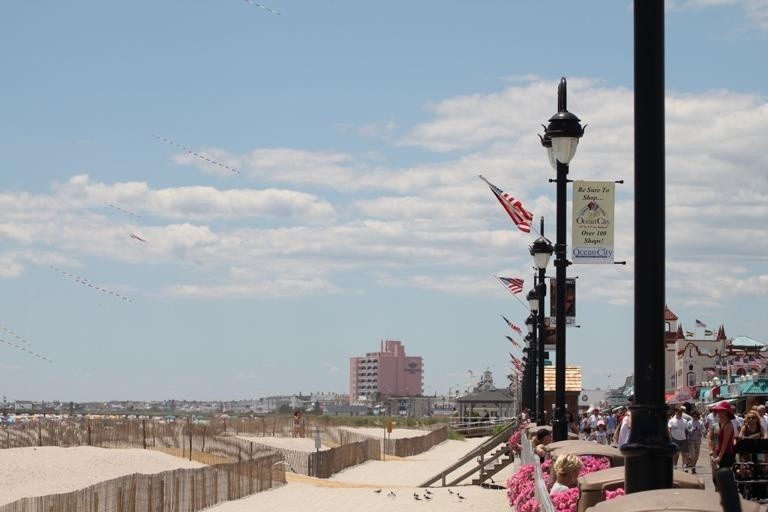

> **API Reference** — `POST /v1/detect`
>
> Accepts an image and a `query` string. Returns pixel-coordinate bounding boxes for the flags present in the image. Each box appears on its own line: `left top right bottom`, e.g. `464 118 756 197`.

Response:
504 317 520 333
704 330 713 336
508 353 521 387
498 277 524 294
489 184 533 233
506 336 518 346
696 320 707 328
687 332 694 337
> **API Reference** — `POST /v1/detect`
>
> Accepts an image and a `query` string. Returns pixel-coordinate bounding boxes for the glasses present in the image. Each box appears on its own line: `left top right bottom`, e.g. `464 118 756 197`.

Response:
748 416 756 420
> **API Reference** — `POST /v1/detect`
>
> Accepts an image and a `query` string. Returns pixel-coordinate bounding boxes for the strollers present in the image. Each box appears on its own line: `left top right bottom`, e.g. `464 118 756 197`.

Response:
732 434 768 507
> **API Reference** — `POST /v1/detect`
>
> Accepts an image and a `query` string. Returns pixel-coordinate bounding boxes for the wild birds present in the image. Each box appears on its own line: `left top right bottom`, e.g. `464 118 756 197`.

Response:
413 491 419 496
491 478 495 483
424 489 432 495
448 488 454 495
414 496 422 501
456 492 464 501
374 488 382 493
423 494 431 499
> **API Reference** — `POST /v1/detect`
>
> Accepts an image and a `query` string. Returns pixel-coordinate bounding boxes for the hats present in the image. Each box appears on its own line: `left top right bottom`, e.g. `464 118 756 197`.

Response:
597 420 605 426
711 402 736 420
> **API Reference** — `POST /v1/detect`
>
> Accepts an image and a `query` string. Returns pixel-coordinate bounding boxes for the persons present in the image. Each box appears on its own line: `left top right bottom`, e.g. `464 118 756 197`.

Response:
520 401 768 496
294 411 303 437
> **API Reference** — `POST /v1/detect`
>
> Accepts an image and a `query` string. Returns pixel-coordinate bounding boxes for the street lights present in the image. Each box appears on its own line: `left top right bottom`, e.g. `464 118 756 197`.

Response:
381 389 469 431
530 212 555 425
542 77 587 448
518 285 538 421
